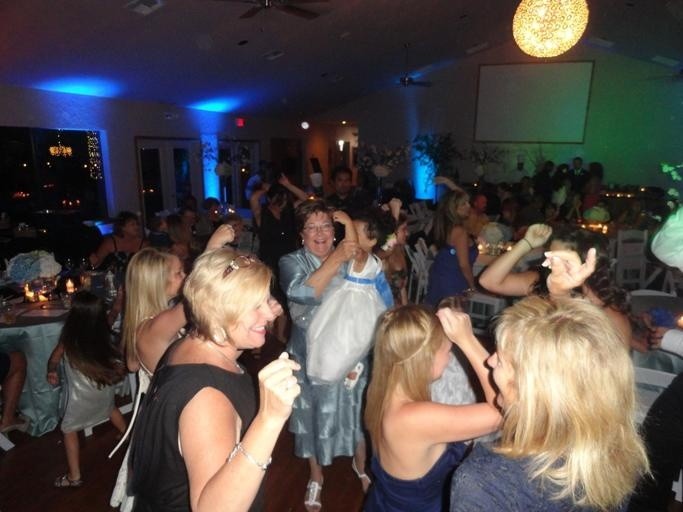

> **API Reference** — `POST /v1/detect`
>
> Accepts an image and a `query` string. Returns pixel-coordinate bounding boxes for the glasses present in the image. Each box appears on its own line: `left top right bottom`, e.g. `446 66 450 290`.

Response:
302 224 334 236
223 254 263 279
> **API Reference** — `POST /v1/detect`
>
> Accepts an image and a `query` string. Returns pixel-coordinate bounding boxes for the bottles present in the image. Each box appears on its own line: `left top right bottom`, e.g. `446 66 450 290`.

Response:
104 269 117 297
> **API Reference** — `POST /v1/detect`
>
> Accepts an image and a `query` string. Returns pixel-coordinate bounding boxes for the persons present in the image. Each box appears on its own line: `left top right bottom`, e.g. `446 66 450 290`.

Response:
0 158 682 511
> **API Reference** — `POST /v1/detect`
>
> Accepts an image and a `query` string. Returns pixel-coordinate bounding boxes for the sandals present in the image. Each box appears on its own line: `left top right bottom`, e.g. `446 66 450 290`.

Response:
344 361 364 391
349 457 370 492
54 473 83 488
305 474 323 512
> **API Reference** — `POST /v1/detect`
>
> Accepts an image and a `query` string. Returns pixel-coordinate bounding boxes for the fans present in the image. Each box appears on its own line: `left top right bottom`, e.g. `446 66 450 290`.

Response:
234 0 323 21
393 42 434 90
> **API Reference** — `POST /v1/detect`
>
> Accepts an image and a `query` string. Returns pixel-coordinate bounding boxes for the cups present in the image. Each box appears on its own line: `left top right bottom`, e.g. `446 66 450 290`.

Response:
63 256 88 272
60 292 73 309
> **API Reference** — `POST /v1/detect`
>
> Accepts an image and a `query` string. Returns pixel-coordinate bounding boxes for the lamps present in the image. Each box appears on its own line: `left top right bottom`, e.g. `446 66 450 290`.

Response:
48 128 71 159
510 0 589 60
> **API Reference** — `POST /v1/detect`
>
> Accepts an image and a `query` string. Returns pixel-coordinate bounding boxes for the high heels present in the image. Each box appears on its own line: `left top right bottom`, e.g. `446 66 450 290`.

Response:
3 414 32 439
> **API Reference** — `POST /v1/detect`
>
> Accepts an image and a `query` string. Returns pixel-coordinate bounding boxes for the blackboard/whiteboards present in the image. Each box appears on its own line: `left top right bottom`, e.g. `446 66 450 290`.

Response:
472 59 595 145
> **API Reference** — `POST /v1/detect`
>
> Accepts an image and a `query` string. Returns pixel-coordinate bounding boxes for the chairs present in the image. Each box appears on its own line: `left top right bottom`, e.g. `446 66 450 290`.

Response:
0 219 683 455
